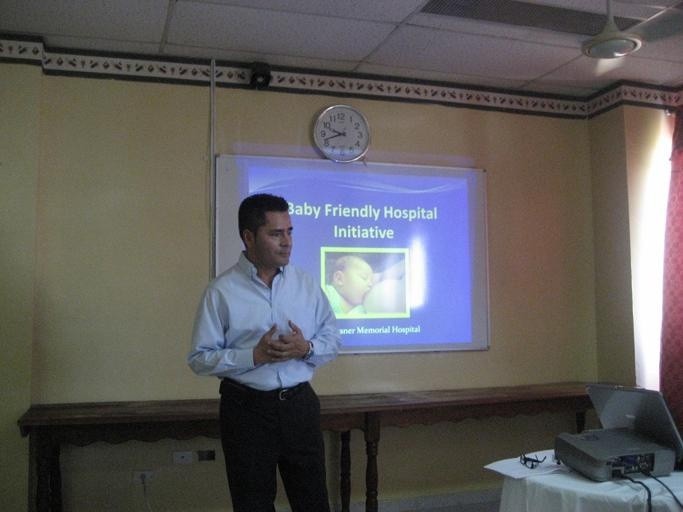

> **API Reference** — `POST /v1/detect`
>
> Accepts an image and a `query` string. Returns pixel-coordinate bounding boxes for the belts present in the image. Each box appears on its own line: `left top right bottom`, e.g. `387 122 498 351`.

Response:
225 377 309 401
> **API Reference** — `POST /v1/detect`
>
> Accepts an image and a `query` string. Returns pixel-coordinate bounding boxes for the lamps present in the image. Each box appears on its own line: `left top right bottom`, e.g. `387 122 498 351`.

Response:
580 0 642 61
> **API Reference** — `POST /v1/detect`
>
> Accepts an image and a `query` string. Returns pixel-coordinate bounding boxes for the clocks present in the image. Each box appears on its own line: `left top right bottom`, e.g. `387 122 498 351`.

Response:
313 104 373 164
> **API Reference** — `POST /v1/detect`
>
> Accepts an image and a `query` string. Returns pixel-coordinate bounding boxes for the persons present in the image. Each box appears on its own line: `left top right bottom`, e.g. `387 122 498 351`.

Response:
326 255 374 315
187 193 342 512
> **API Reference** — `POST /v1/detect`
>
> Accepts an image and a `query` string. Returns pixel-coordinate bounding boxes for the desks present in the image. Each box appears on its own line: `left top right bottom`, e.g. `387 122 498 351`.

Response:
17 380 624 510
505 448 682 512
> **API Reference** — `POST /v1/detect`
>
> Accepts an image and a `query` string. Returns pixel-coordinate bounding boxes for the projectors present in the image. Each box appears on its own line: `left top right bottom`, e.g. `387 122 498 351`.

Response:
554 426 677 482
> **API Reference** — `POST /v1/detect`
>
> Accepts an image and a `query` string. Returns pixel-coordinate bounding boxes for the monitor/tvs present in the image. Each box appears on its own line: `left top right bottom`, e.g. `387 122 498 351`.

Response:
585 383 683 471
